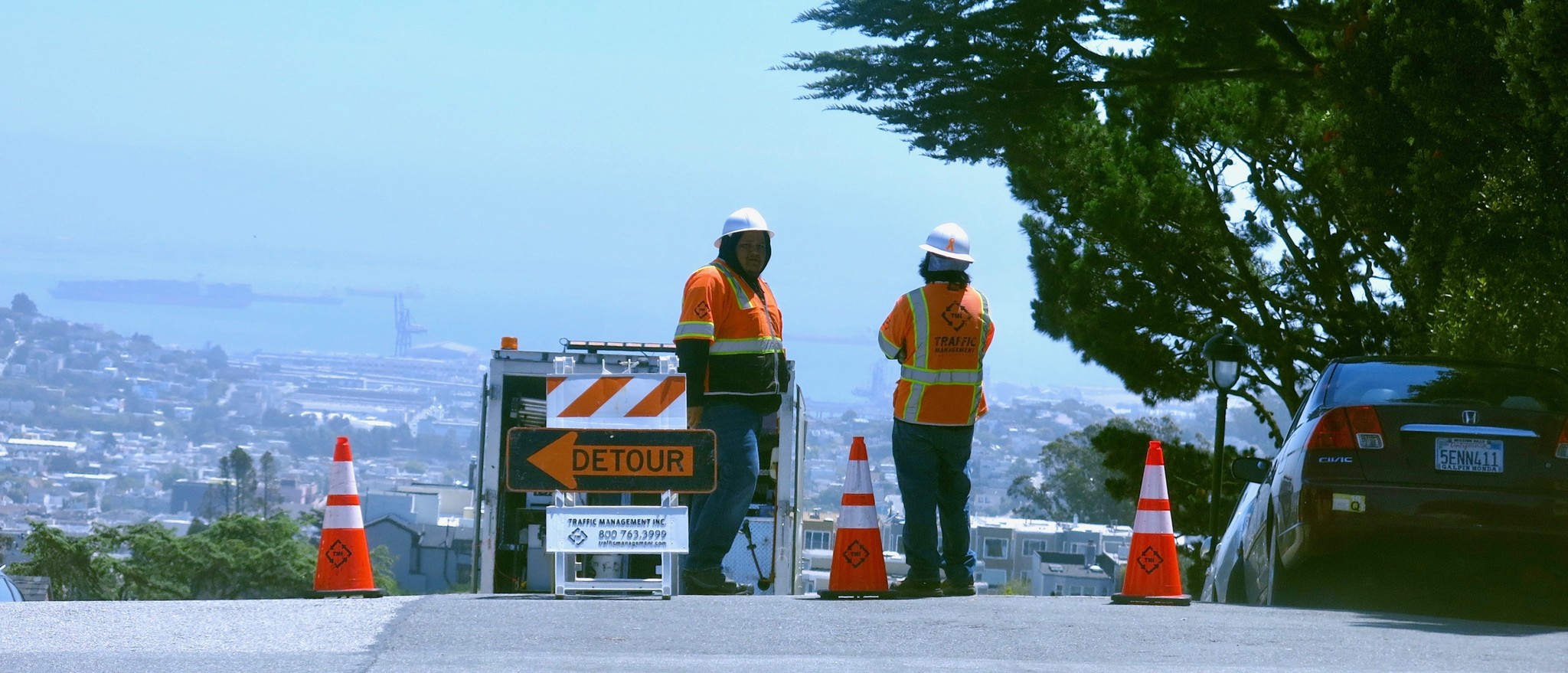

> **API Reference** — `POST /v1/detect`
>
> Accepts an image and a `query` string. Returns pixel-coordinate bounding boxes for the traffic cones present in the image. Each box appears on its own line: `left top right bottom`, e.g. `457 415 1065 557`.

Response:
307 436 385 600
817 436 900 600
1110 440 1193 606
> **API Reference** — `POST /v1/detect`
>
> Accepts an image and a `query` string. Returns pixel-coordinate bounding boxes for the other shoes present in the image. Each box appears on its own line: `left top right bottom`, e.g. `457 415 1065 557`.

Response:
941 578 976 595
682 569 754 596
890 578 943 597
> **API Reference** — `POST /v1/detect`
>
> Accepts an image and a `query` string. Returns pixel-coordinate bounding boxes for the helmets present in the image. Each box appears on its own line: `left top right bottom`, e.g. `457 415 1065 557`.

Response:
713 208 775 248
919 223 975 263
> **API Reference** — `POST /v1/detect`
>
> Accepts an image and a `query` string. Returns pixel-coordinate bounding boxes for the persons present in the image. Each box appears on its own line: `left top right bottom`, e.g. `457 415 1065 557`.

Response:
878 221 995 599
672 208 789 595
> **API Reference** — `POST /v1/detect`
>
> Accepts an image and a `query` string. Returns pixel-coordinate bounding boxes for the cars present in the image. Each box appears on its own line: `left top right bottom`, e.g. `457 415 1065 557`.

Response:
1200 350 1568 628
0 570 26 603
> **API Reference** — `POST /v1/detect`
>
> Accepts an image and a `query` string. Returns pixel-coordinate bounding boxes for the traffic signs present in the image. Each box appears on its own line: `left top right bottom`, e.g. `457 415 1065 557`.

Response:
504 425 718 494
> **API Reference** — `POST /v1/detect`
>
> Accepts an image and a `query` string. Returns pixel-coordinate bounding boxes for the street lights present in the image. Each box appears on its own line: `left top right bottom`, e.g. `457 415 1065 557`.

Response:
1197 323 1250 562
